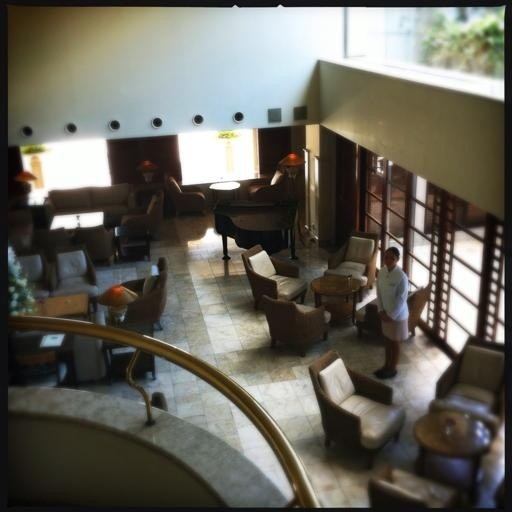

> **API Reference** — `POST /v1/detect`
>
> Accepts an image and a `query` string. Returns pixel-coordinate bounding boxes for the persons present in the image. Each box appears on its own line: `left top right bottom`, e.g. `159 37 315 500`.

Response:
374 247 410 380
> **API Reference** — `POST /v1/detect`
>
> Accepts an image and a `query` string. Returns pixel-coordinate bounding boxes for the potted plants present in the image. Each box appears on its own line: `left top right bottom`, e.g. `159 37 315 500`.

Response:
217 131 240 170
22 142 46 188
